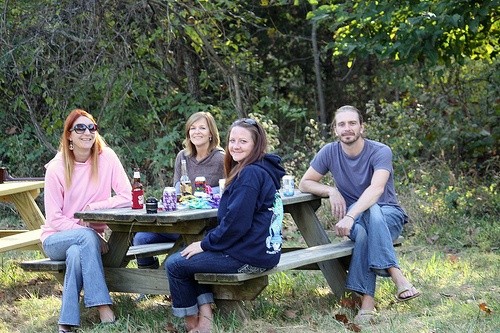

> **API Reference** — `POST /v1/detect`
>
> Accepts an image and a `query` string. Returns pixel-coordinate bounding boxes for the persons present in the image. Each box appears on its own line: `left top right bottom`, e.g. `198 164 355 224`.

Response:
39 109 132 333
300 105 422 324
166 118 286 333
133 113 226 302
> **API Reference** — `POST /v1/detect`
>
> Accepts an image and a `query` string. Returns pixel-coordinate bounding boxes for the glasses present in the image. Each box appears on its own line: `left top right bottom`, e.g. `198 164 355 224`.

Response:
233 118 261 135
69 123 98 134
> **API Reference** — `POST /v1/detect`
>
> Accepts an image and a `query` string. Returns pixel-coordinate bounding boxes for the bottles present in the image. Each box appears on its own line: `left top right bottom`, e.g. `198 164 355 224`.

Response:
146 197 158 213
179 159 191 199
132 167 143 209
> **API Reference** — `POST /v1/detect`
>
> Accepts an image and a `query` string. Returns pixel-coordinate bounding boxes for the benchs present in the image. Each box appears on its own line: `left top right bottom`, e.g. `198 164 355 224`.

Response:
0 228 45 255
19 243 175 274
194 241 357 285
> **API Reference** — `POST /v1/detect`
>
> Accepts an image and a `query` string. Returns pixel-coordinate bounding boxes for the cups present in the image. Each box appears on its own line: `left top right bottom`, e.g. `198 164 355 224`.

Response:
219 178 226 198
0 166 9 183
162 187 177 210
194 176 206 193
282 175 294 197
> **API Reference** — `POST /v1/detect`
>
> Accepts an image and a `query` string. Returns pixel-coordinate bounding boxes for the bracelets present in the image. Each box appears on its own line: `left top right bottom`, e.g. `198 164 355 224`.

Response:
344 214 357 222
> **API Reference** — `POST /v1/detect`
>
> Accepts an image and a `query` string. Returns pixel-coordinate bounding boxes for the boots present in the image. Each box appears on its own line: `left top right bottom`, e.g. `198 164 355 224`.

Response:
134 257 159 302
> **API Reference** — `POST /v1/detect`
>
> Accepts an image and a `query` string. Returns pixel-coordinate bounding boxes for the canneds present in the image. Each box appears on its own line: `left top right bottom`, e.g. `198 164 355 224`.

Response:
282 175 294 197
218 179 226 198
194 176 206 196
162 187 177 212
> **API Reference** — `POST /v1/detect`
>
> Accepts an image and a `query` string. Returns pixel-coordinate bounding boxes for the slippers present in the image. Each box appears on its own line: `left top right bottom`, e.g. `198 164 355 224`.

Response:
58 330 73 333
394 284 421 302
101 315 117 327
354 306 377 324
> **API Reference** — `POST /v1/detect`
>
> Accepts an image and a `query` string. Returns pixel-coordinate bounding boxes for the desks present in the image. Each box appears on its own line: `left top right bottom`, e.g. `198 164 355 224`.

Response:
0 179 66 296
74 189 348 304
